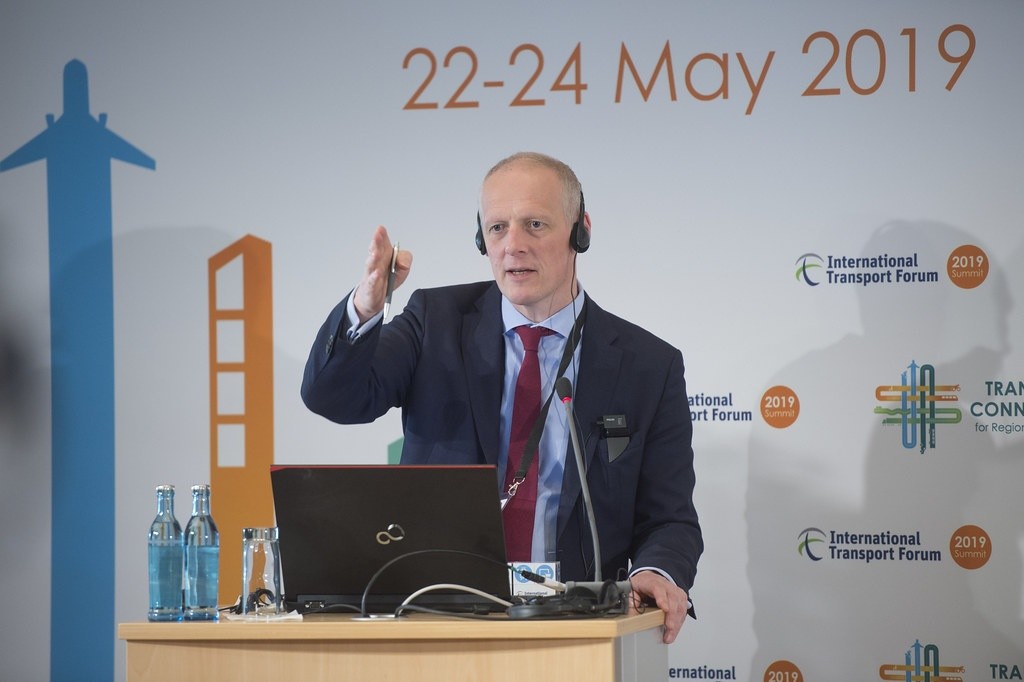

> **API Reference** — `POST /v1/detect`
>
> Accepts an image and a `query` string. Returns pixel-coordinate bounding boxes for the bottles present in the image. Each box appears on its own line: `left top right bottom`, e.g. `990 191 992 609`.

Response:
147 484 183 622
182 484 220 620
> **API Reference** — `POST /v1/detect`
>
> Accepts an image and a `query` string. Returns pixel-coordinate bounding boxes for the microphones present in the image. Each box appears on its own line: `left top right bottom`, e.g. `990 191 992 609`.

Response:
555 376 632 601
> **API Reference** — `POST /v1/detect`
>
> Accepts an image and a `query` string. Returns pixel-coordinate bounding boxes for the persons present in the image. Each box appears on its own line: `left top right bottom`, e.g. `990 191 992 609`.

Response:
300 151 703 644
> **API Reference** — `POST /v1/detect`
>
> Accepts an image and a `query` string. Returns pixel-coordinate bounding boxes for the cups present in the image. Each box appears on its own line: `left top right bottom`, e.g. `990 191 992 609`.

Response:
242 527 282 616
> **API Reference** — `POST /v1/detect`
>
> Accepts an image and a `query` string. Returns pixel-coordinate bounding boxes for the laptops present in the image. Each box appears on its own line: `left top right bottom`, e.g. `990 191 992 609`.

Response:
270 462 565 614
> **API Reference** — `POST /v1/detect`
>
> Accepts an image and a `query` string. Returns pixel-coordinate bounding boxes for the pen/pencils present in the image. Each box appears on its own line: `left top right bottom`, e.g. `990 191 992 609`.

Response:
383 240 400 321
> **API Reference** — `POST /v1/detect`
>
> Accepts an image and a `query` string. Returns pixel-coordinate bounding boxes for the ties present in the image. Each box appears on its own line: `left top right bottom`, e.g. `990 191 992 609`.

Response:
502 325 558 562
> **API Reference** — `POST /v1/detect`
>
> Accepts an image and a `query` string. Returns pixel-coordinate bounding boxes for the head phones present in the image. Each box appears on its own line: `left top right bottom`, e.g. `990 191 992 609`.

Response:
503 580 622 619
475 190 589 255
